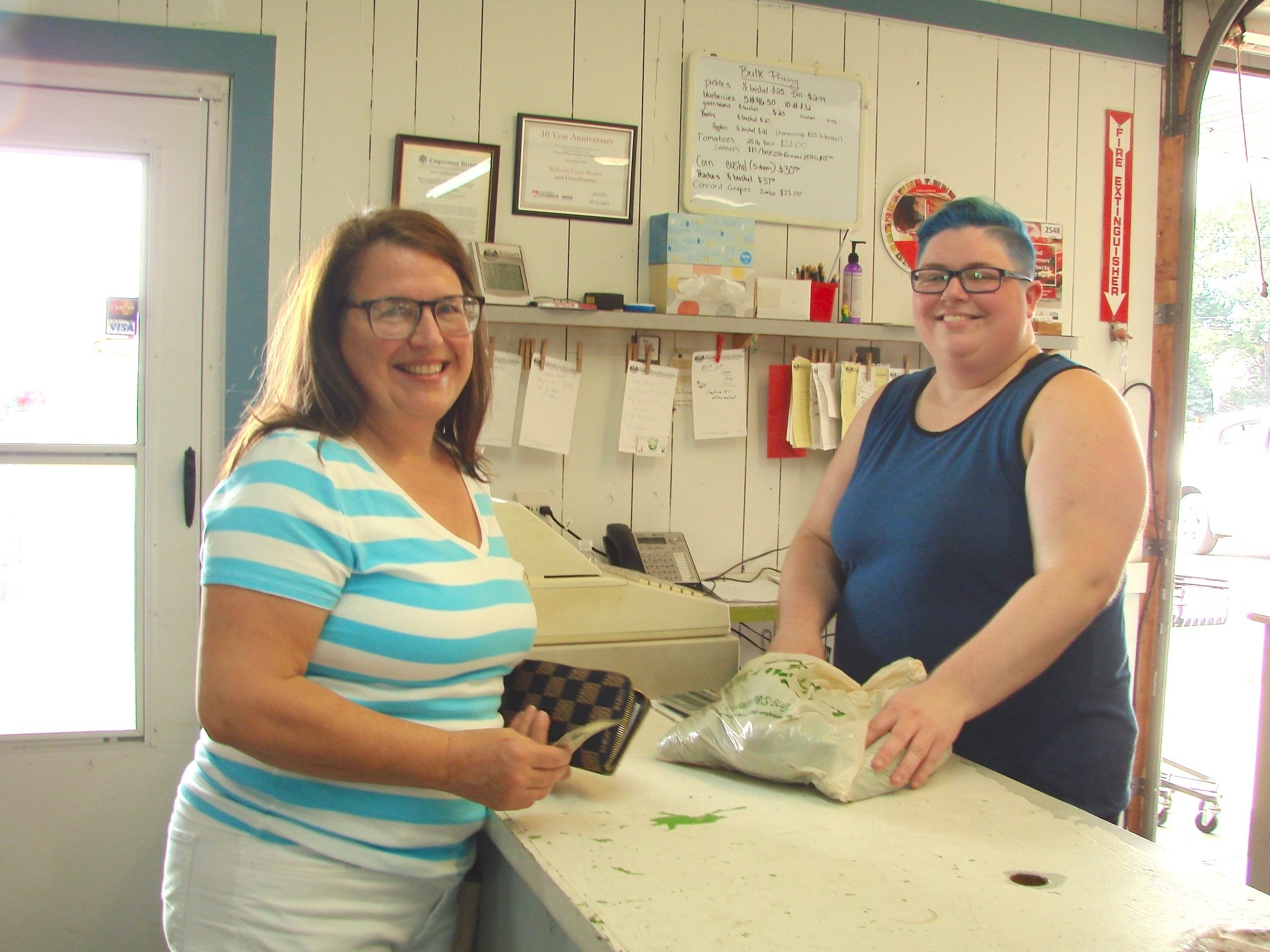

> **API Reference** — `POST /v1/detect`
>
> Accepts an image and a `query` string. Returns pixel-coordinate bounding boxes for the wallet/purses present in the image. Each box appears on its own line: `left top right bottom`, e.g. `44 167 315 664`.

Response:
499 659 651 776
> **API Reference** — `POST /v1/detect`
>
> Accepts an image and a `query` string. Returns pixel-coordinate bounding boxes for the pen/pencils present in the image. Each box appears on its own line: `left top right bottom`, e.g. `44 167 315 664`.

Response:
831 274 838 282
797 262 824 283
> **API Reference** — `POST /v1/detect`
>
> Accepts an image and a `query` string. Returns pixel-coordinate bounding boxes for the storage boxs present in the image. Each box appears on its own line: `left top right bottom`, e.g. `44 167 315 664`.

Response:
648 212 755 319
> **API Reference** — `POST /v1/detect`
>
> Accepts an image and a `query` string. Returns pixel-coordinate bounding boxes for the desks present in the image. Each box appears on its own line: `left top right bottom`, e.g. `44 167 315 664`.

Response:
471 682 1270 952
700 573 780 620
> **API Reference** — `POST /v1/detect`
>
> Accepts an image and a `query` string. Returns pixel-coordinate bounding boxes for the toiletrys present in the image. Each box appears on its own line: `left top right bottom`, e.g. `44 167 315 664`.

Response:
841 239 866 324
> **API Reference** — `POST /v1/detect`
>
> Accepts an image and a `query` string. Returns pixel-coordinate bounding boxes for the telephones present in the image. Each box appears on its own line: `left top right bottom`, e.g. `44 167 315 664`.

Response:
603 524 702 591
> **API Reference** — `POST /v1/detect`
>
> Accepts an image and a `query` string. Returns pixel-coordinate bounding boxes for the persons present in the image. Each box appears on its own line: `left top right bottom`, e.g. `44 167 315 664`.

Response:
767 198 1147 826
161 210 572 952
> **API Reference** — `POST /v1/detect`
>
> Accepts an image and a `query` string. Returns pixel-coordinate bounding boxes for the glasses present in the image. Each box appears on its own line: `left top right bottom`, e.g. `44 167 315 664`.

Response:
911 266 1033 294
344 295 485 340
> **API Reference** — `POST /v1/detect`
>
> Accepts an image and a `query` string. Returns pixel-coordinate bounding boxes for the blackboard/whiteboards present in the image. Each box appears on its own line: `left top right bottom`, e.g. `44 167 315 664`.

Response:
681 52 868 232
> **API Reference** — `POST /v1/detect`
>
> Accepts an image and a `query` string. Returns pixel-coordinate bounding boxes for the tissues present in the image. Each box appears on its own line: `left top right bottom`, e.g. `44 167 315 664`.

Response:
649 264 755 319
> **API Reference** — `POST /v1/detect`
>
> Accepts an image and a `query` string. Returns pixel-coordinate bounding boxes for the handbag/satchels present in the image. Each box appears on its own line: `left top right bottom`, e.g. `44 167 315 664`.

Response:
656 653 953 802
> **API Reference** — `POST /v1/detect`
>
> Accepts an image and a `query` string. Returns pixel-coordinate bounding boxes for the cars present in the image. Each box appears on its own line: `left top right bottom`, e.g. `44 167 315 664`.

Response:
1177 406 1270 559
